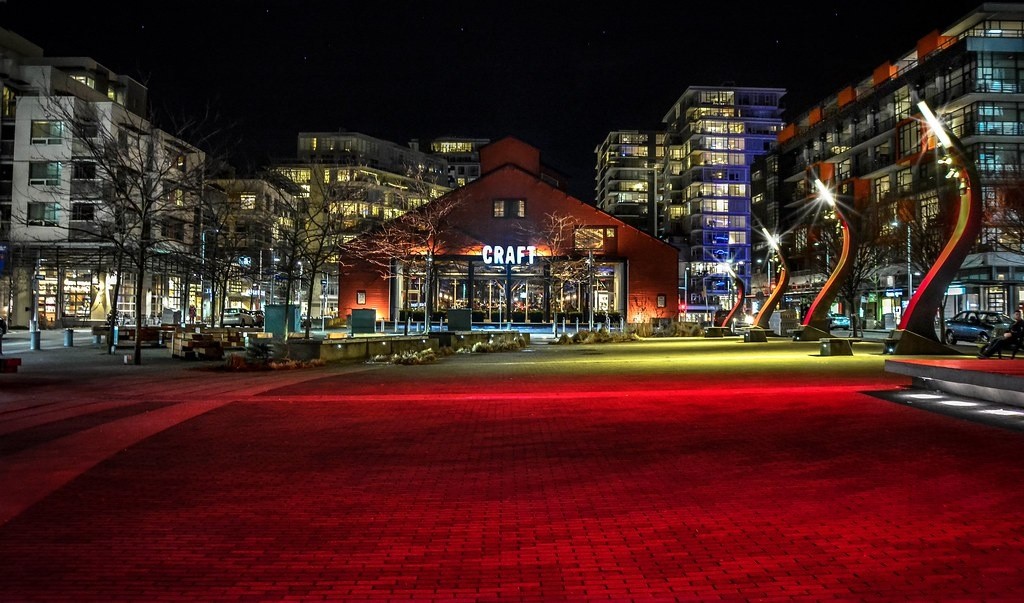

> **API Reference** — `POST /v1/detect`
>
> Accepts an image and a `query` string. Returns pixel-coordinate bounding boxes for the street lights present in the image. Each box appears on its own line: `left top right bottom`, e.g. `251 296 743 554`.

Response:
586 248 595 333
757 259 771 298
815 240 830 275
684 267 690 322
321 279 327 332
201 228 219 322
259 247 274 310
891 222 912 300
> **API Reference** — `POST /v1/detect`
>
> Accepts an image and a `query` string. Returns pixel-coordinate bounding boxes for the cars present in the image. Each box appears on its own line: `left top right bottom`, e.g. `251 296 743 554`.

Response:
943 309 1017 345
0 316 7 338
733 315 750 328
250 310 264 328
302 315 312 321
220 308 254 328
830 313 851 330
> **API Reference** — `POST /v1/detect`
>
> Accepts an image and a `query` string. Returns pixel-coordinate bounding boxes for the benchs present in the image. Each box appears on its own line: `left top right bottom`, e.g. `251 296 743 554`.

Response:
430 330 520 353
704 327 730 338
285 335 430 363
744 329 774 342
818 337 862 356
